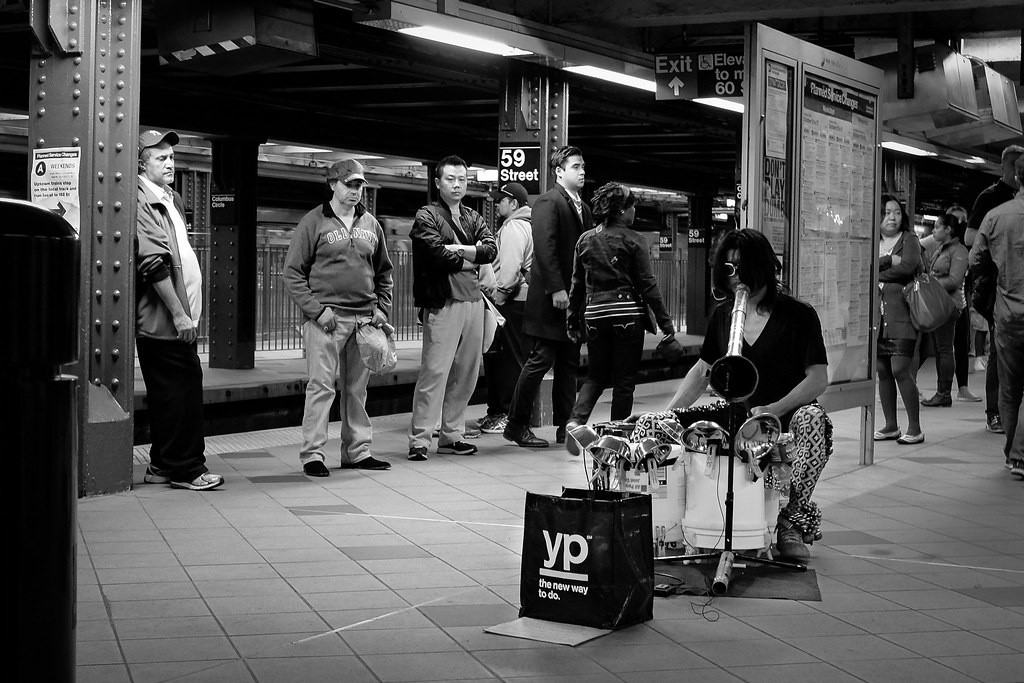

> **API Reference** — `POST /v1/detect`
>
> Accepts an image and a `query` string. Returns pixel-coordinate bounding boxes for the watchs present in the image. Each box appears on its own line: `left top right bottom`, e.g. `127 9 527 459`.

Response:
457 244 466 257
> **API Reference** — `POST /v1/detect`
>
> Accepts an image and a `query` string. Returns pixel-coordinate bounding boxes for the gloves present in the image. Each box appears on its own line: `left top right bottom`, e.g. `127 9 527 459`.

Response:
651 333 686 358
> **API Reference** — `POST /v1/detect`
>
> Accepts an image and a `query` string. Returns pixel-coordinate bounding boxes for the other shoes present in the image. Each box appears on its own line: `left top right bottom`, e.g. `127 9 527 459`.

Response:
303 461 329 477
341 456 392 470
974 356 986 371
565 421 582 456
1004 457 1014 469
897 432 924 445
872 429 901 440
1010 460 1024 476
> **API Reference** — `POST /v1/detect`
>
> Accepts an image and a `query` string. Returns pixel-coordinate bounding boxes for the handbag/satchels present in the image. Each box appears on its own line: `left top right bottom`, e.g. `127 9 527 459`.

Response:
902 273 956 333
645 303 658 335
481 308 505 353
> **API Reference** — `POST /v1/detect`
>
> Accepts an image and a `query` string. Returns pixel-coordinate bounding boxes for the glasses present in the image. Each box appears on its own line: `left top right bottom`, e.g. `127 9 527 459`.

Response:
723 261 741 277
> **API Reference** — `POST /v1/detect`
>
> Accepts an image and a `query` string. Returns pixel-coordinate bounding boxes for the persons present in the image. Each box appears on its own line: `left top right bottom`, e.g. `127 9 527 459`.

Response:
874 144 1024 479
409 145 676 462
628 229 835 567
134 130 225 491
282 159 394 476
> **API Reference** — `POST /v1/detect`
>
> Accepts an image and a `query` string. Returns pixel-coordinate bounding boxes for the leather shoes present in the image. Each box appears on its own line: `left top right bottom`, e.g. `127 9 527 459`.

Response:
503 423 549 447
921 393 952 408
555 428 566 443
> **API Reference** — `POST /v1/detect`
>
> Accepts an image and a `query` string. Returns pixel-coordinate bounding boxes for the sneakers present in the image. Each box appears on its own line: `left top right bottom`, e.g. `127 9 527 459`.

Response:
170 470 224 492
776 527 810 559
437 441 478 455
434 423 481 439
407 446 427 460
957 386 983 402
475 413 508 434
985 414 1004 433
143 462 172 483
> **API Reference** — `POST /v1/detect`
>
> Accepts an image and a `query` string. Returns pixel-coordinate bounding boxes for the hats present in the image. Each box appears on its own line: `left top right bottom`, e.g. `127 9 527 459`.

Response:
328 158 368 185
488 182 529 203
139 129 180 155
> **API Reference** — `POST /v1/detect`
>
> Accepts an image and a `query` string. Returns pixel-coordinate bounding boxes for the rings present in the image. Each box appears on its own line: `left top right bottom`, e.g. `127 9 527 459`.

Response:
325 326 328 331
378 323 383 327
